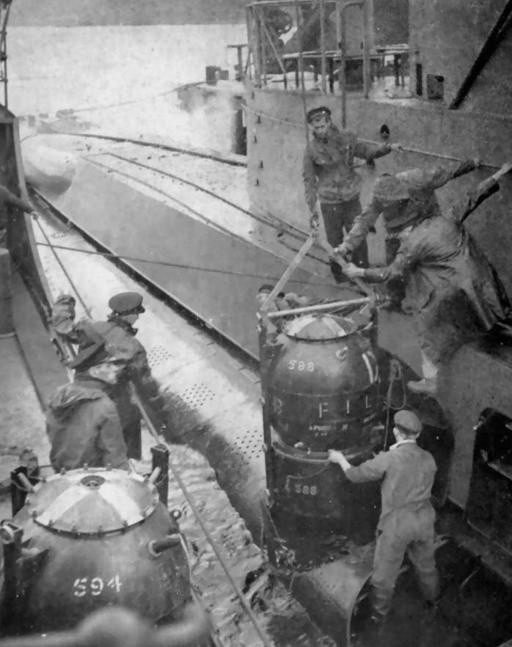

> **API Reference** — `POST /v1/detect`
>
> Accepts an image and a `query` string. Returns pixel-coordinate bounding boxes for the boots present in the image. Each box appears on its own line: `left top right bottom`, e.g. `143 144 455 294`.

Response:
407 374 437 394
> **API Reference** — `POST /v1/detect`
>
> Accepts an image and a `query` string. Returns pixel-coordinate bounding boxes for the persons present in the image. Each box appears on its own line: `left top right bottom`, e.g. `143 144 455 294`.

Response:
43 339 129 477
332 157 492 312
344 160 511 397
301 105 404 282
51 281 171 461
324 409 441 627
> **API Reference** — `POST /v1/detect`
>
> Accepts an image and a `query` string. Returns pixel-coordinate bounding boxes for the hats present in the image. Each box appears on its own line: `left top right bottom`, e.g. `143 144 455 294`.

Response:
304 103 331 122
109 291 145 315
68 339 118 372
386 208 417 233
393 409 422 434
